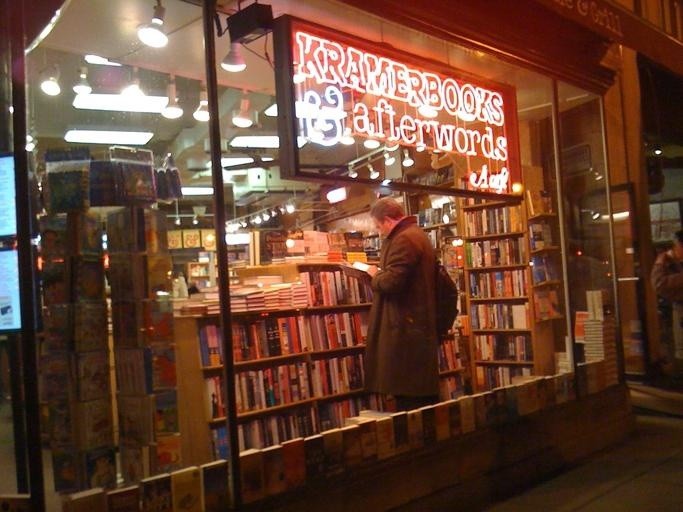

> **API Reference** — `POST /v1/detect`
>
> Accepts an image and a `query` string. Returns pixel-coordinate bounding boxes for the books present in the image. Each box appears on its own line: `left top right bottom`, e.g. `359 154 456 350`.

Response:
338 260 381 280
461 179 534 393
69 373 577 510
438 338 463 401
39 164 179 492
189 228 387 460
525 188 562 321
577 317 618 395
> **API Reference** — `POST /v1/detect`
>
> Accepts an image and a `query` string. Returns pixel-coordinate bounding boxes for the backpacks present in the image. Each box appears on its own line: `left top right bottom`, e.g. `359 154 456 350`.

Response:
434 255 459 336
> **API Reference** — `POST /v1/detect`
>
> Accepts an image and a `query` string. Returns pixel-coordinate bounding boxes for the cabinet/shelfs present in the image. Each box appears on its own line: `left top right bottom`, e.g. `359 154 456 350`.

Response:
427 205 558 392
170 248 419 466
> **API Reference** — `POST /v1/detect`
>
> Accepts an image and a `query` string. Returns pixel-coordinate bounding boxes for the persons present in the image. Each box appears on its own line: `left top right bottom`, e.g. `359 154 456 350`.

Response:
651 231 683 379
362 196 441 415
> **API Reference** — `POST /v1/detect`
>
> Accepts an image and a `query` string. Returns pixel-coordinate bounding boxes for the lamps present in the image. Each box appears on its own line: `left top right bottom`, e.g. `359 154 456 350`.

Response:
220 42 248 73
39 54 358 153
205 126 428 232
137 8 169 48
